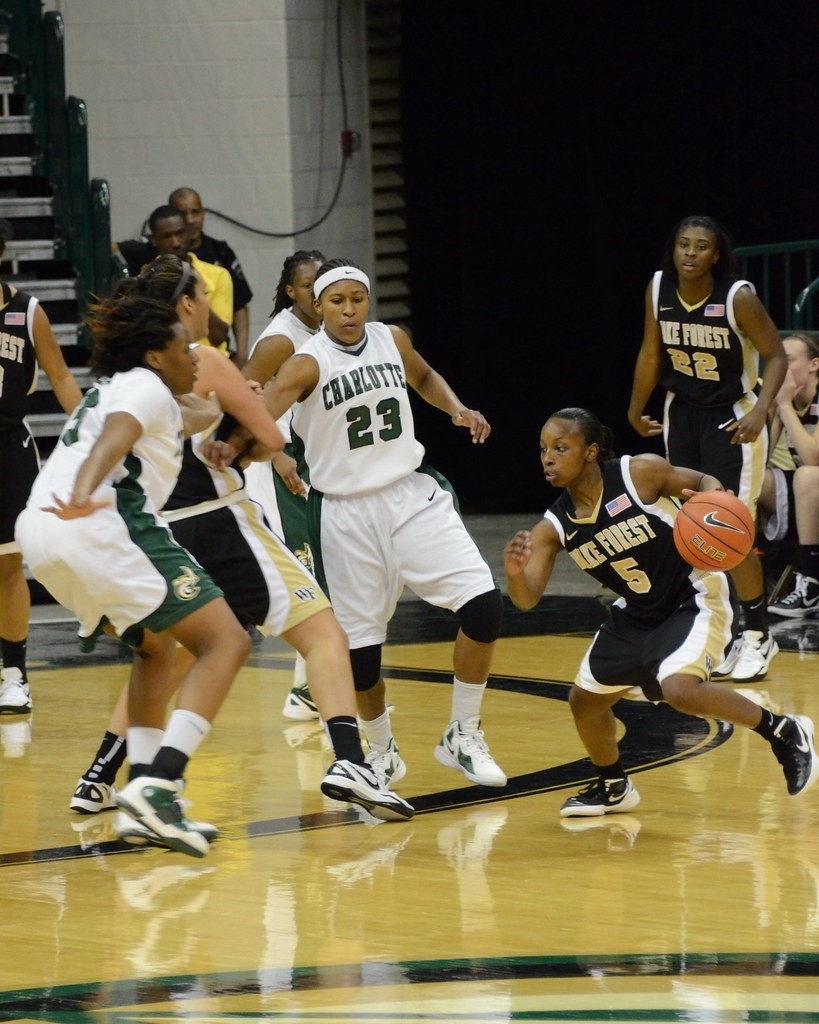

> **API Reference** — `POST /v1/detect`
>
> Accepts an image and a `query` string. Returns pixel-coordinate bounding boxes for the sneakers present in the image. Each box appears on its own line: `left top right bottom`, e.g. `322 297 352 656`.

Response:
435 721 507 788
321 759 415 821
559 776 642 817
70 777 118 814
282 684 394 721
771 714 817 798
360 737 407 787
768 574 819 618
114 777 219 858
708 629 779 682
0 667 32 713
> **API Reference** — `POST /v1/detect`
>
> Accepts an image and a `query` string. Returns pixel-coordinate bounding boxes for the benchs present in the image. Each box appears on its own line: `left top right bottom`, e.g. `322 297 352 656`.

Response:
1 34 100 606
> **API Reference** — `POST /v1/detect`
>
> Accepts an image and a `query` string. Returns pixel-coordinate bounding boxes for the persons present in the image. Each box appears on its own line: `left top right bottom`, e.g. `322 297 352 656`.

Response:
503 406 819 819
756 330 819 618
627 217 789 684
0 186 507 856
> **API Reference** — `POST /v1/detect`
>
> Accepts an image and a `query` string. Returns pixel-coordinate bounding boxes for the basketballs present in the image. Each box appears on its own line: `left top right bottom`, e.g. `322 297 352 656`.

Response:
674 490 755 571
